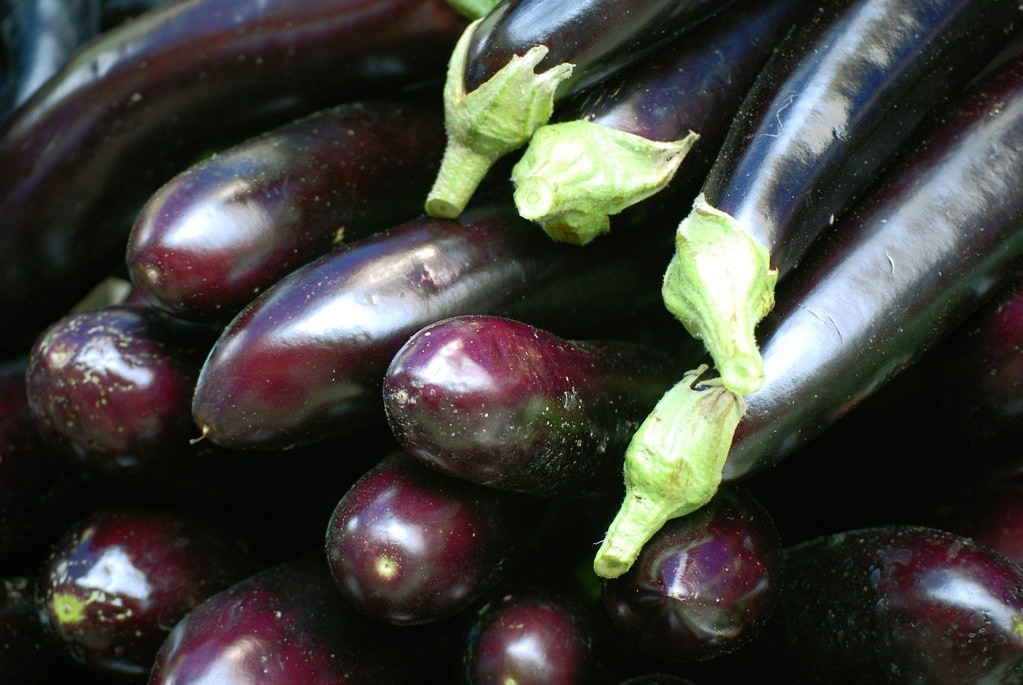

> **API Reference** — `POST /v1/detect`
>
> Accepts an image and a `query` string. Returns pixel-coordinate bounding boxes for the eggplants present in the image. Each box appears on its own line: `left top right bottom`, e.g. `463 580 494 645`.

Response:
0 0 1023 685
